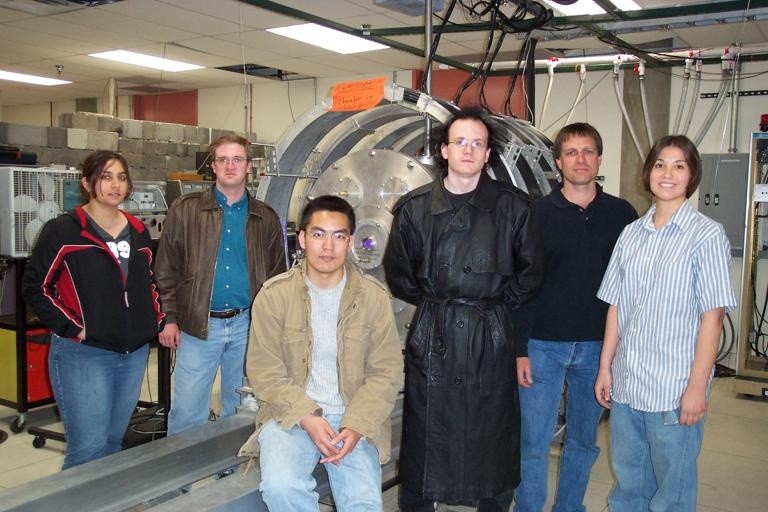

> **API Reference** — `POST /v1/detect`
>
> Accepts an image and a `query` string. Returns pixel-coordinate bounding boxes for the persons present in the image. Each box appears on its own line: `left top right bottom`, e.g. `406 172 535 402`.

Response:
512 123 639 512
593 135 738 512
20 150 167 470
382 107 545 512
154 135 286 494
245 195 404 512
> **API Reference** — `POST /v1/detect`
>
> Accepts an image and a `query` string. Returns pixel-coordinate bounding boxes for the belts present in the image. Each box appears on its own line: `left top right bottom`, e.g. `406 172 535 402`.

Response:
209 308 248 319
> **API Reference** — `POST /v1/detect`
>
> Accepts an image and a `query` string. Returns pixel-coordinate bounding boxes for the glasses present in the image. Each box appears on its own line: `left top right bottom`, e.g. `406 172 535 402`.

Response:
446 139 489 150
306 231 350 243
214 158 249 164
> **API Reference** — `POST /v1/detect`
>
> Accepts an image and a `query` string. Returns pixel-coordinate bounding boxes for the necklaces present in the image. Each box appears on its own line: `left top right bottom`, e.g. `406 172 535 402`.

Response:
104 214 117 232
